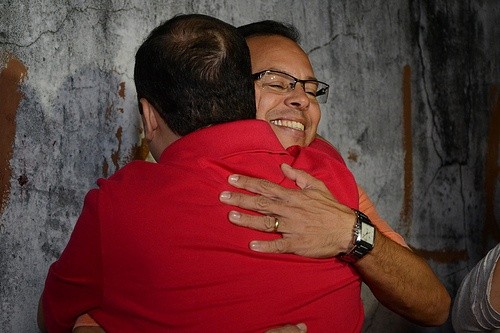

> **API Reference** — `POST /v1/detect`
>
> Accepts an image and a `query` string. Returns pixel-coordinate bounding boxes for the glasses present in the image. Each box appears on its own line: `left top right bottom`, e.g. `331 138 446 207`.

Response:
252 70 329 104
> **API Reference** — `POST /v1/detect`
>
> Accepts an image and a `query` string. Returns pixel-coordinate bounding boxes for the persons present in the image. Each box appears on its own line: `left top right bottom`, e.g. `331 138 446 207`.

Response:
450 244 500 333
72 19 451 333
36 14 359 333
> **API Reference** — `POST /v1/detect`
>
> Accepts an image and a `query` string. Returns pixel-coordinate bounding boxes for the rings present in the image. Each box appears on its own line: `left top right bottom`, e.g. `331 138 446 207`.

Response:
274 216 279 233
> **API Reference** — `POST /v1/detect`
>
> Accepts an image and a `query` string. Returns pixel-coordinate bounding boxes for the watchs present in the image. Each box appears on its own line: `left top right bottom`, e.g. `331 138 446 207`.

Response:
338 207 376 266
